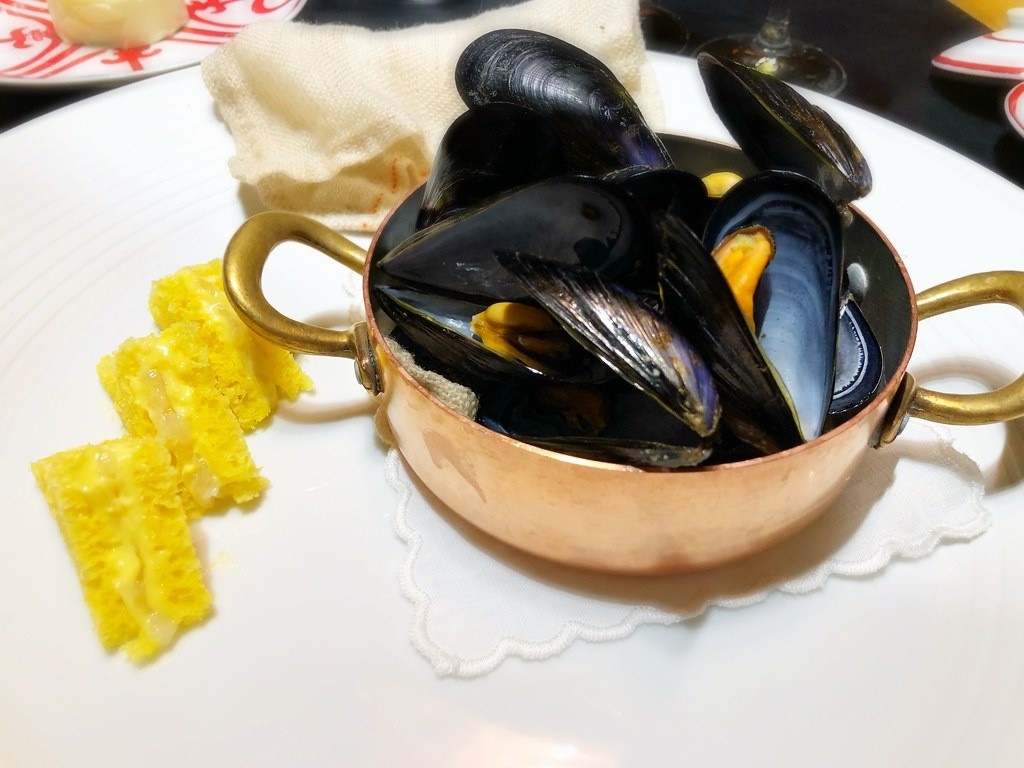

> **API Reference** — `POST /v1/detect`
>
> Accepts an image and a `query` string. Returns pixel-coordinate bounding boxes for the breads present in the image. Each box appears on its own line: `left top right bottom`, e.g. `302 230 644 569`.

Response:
30 261 314 667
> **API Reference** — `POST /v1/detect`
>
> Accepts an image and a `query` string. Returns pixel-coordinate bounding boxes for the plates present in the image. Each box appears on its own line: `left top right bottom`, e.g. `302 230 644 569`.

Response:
0 0 310 87
0 47 1024 768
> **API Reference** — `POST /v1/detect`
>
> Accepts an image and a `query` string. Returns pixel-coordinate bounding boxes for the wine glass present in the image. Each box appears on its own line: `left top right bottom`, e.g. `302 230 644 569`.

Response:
693 0 846 100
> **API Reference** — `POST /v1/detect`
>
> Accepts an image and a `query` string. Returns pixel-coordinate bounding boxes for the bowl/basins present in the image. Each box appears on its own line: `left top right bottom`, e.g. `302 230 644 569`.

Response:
222 131 1024 579
